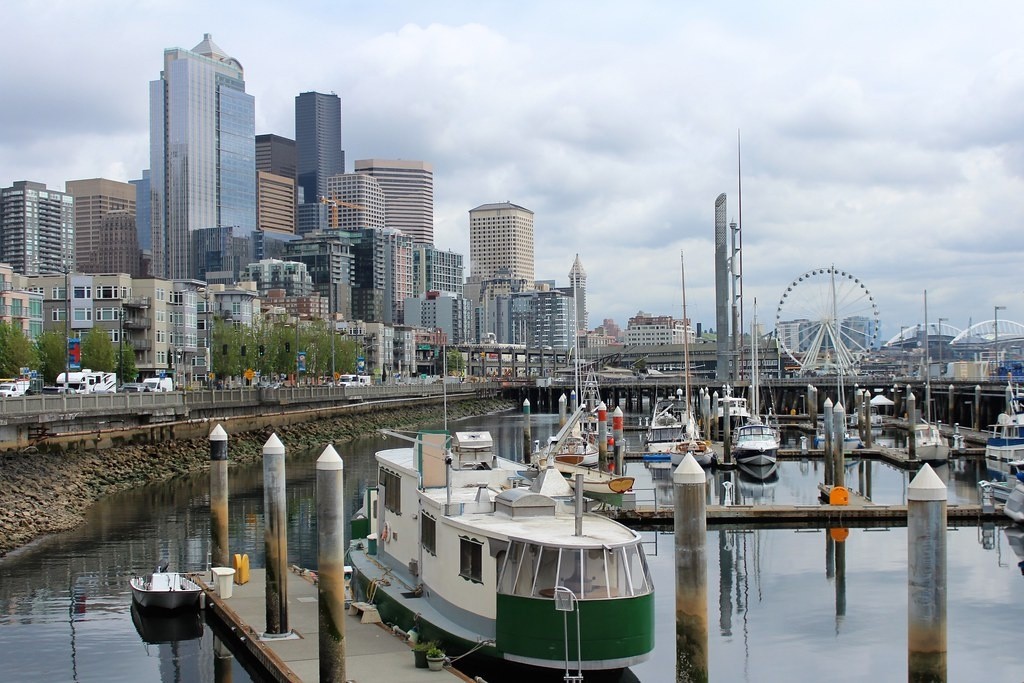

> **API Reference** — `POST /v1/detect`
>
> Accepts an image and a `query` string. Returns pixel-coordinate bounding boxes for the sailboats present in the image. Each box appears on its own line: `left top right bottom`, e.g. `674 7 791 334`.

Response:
644 249 780 465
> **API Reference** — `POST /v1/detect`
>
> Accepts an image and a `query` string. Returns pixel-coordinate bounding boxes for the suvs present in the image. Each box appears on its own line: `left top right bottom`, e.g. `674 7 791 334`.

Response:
41 387 78 395
123 386 151 392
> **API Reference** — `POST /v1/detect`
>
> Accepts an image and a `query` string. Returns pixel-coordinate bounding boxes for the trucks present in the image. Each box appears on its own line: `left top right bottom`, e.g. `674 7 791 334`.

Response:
337 375 371 386
142 378 173 392
0 378 30 397
56 369 116 394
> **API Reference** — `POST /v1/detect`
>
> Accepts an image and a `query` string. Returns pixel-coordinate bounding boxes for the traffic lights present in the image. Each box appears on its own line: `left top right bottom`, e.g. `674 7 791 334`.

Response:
259 345 264 356
285 342 290 352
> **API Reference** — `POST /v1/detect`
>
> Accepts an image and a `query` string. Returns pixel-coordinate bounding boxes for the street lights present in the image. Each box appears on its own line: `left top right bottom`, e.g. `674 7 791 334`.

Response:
938 317 949 365
994 306 1006 367
900 326 910 372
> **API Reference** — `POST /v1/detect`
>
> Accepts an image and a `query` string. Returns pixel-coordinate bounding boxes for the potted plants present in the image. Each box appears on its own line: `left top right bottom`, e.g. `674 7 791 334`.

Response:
411 641 435 668
425 648 445 671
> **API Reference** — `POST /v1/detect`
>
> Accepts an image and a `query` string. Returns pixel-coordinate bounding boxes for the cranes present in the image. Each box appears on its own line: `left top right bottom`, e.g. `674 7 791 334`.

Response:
320 188 366 229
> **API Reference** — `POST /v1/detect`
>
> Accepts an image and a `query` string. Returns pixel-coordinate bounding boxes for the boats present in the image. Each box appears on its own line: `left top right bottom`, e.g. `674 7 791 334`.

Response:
906 425 949 460
814 406 882 450
346 344 655 671
130 560 203 609
977 381 1024 521
